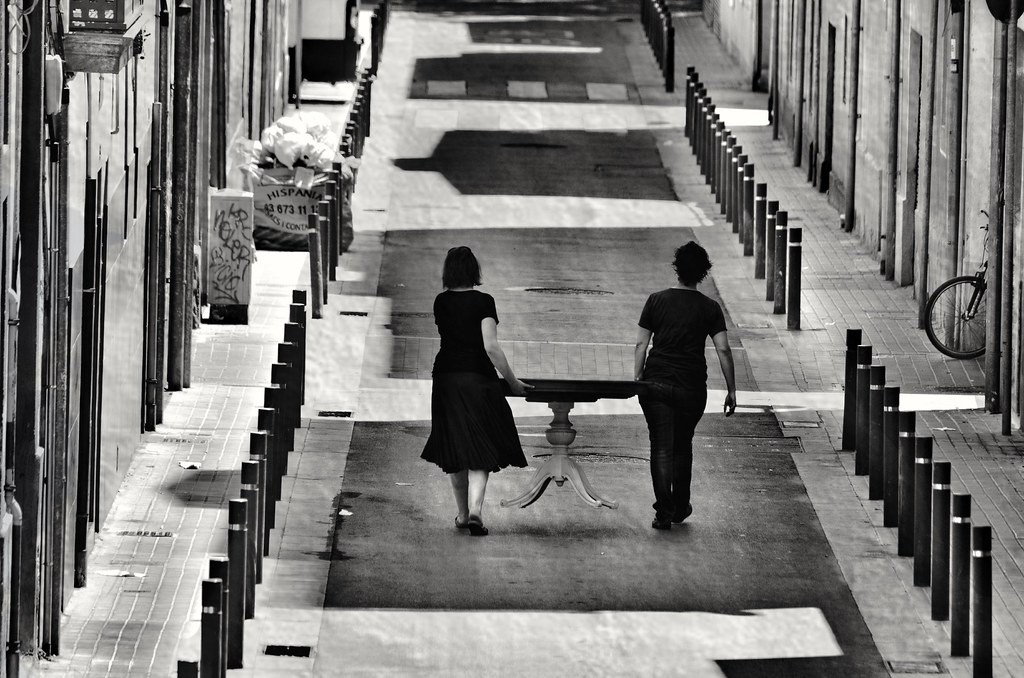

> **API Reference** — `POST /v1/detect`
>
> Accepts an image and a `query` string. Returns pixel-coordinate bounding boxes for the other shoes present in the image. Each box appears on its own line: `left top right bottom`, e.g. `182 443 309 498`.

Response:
671 503 692 523
652 519 672 531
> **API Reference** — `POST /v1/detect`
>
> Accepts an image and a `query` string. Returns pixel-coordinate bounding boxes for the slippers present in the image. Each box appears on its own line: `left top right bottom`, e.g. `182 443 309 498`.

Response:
468 520 489 536
455 515 469 528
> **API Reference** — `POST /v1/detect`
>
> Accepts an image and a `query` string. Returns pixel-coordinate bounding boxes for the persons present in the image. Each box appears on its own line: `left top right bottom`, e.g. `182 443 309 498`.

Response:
634 240 736 528
420 245 535 536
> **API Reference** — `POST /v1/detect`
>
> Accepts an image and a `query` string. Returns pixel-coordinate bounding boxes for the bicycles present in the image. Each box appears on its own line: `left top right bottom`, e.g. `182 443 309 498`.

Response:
924 208 992 358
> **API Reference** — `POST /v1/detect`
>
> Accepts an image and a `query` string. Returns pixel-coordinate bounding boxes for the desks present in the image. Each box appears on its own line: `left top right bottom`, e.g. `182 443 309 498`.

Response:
498 377 653 510
